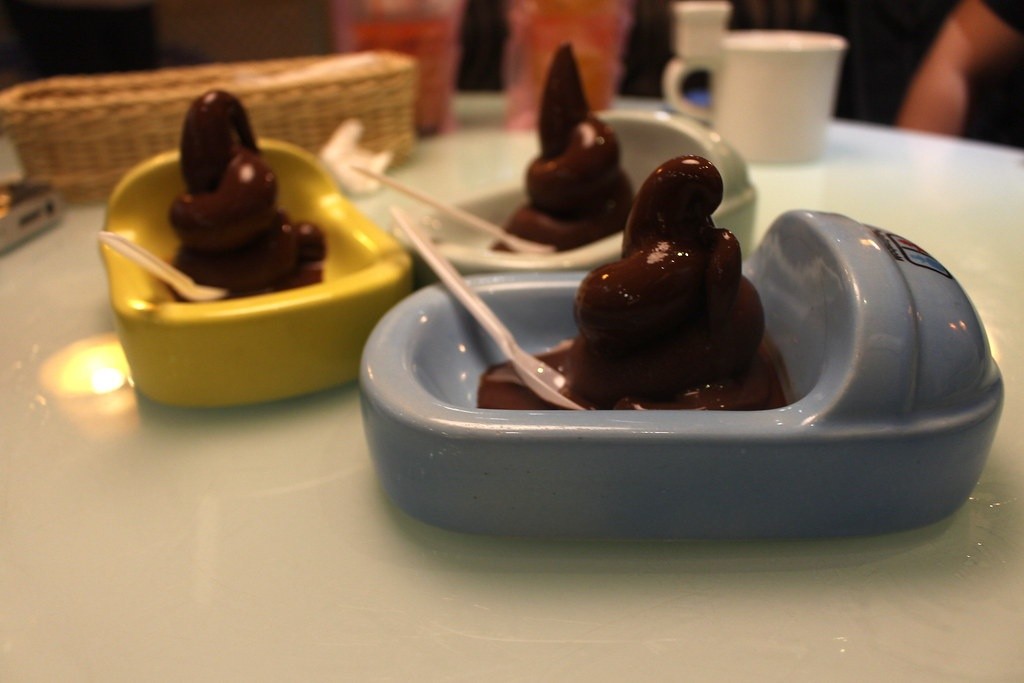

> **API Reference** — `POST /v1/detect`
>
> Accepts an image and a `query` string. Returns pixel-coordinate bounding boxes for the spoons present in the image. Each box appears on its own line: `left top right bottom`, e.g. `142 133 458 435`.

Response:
382 200 597 412
348 159 557 254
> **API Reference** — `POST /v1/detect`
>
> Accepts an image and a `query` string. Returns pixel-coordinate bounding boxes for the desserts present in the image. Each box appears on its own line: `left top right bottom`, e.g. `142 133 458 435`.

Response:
492 39 637 254
566 153 791 410
164 90 327 306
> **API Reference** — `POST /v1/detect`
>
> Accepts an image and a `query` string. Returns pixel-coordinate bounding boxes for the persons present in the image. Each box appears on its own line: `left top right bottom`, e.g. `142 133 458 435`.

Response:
894 0 1024 149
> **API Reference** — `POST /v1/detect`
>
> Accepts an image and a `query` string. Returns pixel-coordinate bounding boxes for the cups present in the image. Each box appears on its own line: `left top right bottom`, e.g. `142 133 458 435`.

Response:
667 33 852 162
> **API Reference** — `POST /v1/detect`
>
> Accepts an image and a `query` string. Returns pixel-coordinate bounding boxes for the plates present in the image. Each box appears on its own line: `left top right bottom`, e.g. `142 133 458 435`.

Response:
357 207 1005 541
106 141 412 414
394 99 760 280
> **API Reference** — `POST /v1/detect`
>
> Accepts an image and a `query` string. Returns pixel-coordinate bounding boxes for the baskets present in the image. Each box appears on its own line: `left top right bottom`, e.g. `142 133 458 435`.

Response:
0 47 418 207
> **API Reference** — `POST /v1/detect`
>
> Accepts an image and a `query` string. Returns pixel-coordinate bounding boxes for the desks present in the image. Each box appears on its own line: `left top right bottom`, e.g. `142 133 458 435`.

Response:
0 88 1024 683
